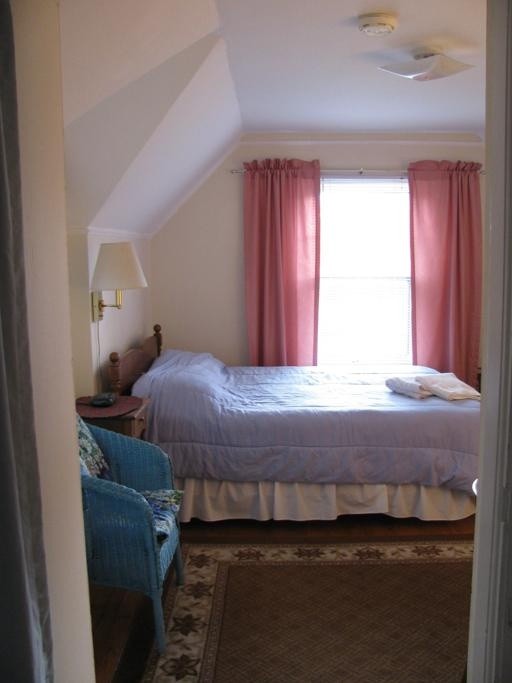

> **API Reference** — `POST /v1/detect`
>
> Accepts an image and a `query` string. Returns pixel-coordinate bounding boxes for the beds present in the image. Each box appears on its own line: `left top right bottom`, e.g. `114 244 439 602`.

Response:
110 324 482 527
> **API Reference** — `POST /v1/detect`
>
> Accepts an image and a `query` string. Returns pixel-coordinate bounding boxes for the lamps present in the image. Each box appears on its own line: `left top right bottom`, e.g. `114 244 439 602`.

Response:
91 241 151 392
376 53 472 81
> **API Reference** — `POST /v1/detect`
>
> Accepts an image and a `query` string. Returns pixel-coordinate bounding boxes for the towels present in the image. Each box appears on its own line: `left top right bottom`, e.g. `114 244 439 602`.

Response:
415 372 481 403
385 375 433 400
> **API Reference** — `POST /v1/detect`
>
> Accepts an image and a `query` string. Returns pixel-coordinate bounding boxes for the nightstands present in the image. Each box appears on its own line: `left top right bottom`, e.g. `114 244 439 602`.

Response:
75 394 151 445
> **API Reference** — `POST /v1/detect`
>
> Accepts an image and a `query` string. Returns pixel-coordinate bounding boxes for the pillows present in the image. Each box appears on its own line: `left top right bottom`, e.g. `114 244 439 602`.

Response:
76 413 111 477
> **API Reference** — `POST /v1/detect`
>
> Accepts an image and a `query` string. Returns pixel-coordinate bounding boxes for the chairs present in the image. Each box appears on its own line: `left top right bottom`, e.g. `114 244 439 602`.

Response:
76 413 185 655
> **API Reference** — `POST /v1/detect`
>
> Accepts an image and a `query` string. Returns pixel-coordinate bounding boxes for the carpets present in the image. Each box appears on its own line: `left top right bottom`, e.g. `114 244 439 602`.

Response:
111 534 474 682
